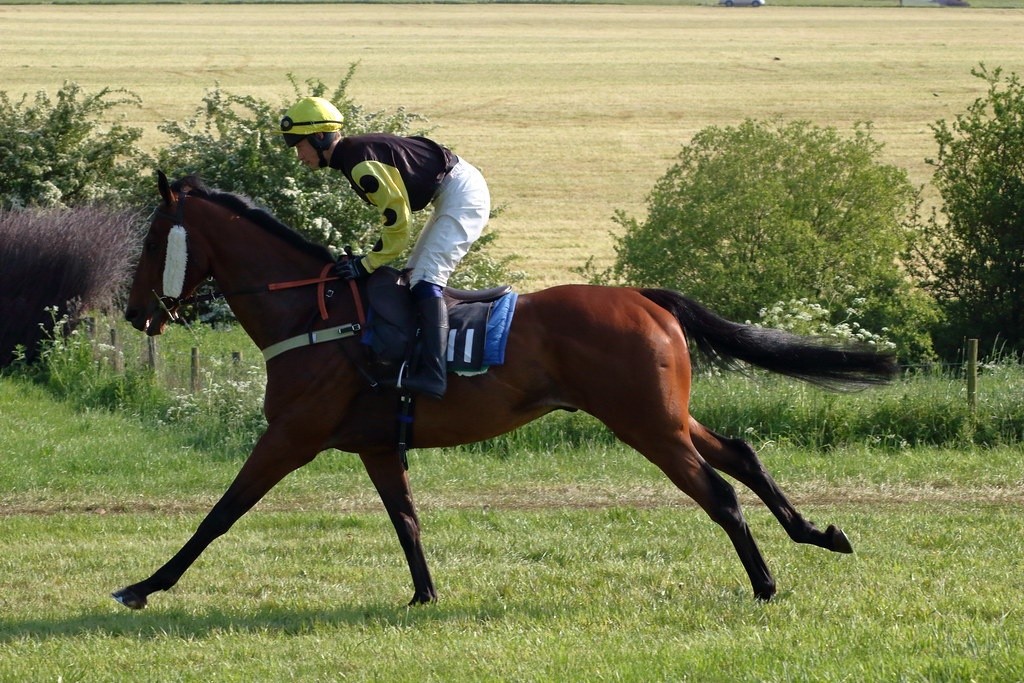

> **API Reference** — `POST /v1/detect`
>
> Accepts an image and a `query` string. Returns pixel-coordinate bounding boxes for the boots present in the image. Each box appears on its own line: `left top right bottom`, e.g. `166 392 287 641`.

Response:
384 297 449 395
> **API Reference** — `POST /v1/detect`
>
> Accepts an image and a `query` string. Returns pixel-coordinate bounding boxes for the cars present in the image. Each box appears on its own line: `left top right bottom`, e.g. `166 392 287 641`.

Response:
718 0 766 7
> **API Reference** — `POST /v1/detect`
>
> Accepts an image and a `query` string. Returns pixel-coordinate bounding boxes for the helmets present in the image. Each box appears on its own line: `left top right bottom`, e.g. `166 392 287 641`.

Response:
270 97 344 148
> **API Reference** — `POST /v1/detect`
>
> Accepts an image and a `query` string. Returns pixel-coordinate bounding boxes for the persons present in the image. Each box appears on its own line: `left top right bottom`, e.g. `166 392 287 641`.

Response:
280 97 491 400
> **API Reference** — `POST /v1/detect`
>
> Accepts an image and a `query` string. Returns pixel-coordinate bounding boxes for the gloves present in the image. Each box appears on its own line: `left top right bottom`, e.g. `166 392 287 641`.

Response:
332 256 374 283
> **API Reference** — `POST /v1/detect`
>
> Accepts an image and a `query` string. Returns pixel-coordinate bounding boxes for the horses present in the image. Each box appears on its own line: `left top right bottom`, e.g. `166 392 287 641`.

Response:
110 167 903 613
